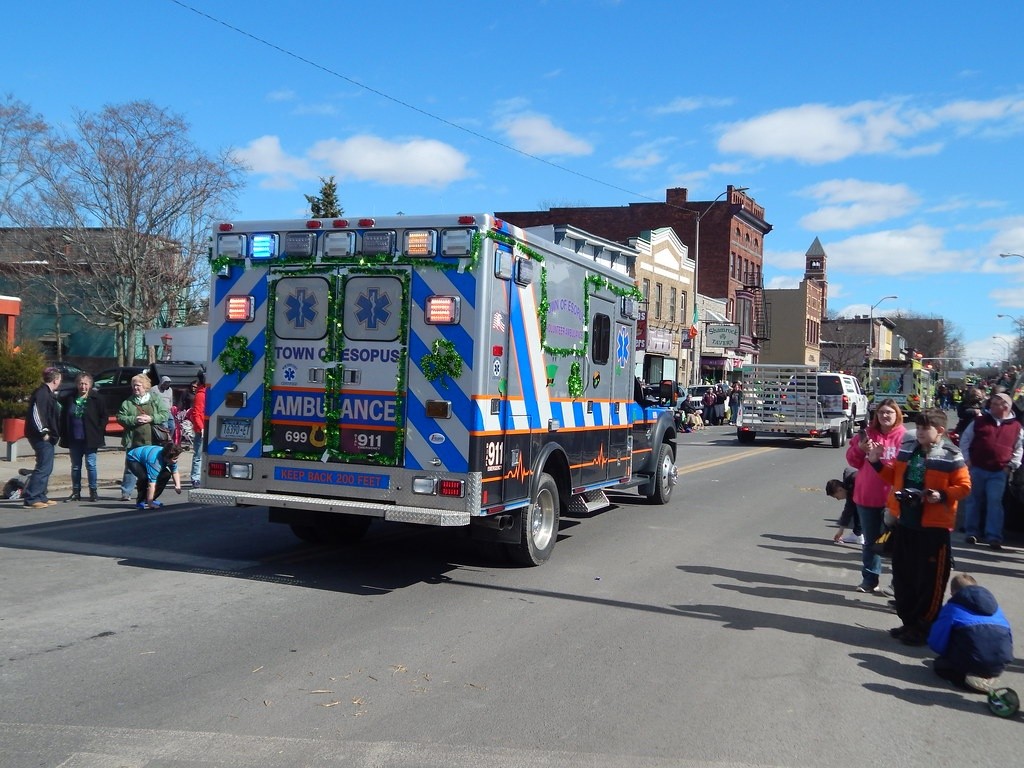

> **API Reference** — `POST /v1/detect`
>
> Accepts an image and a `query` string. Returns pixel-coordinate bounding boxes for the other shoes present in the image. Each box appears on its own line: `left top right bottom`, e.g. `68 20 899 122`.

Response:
991 541 1002 552
967 537 977 544
966 675 1001 694
891 626 910 635
900 631 928 646
192 480 201 488
121 495 132 500
858 571 879 593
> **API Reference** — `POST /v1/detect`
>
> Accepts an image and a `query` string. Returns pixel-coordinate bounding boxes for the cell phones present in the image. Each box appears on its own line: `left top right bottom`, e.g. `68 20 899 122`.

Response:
858 429 869 443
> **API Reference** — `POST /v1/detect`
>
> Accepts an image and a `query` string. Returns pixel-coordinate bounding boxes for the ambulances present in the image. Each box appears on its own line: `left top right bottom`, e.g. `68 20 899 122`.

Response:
187 212 679 566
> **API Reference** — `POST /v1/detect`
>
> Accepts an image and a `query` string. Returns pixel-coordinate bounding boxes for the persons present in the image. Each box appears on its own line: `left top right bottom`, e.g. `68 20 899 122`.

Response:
152 376 174 437
846 400 906 596
59 373 109 503
869 409 973 648
23 367 61 509
958 392 1024 550
117 374 170 501
826 468 865 544
128 443 183 509
766 381 782 410
179 373 206 485
701 380 742 425
928 574 1014 692
937 364 1024 533
675 394 703 432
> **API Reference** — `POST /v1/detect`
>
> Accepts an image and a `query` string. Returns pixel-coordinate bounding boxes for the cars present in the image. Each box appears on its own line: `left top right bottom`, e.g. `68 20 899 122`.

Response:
43 360 90 404
645 384 734 414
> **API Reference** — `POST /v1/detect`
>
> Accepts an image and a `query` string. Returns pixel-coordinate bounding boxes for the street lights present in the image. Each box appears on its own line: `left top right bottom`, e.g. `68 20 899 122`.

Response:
692 187 750 385
991 314 1022 366
869 295 899 380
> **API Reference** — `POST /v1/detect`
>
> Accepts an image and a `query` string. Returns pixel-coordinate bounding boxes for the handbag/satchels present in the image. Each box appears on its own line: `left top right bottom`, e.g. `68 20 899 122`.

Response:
153 424 173 442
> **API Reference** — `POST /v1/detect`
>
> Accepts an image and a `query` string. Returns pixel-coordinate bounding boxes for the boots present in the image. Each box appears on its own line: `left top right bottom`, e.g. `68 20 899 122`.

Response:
89 489 99 502
64 488 81 502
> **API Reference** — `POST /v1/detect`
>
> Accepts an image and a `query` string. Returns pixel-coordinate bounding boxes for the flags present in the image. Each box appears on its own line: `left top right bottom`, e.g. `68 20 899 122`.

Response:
689 305 699 338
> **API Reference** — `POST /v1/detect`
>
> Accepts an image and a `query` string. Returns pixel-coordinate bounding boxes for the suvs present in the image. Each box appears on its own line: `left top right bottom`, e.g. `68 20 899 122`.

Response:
59 360 201 444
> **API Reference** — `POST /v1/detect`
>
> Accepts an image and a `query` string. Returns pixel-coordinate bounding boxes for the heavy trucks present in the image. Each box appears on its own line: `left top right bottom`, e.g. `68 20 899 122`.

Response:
736 373 869 448
868 358 939 420
940 374 982 405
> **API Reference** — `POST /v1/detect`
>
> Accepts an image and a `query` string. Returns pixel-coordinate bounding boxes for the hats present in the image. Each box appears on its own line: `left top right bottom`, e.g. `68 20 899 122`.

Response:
997 392 1013 408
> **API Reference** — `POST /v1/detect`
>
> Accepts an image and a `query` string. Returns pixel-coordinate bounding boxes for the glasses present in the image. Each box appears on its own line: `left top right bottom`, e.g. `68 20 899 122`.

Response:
878 410 896 415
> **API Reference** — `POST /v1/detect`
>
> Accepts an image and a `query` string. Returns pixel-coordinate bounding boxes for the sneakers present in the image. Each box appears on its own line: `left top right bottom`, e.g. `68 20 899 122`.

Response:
153 500 164 506
844 532 865 545
138 501 147 507
23 502 48 509
45 500 57 506
